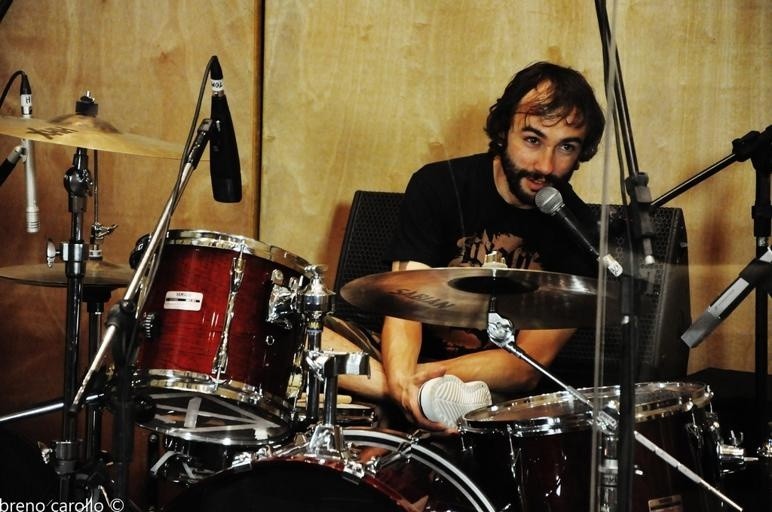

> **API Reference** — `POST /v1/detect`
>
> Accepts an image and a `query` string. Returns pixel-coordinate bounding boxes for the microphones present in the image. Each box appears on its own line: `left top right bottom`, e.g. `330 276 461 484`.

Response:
20 75 41 234
535 186 624 278
210 57 242 203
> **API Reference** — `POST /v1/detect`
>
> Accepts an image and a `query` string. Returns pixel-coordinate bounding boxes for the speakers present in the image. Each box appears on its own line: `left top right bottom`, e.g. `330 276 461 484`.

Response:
319 190 691 396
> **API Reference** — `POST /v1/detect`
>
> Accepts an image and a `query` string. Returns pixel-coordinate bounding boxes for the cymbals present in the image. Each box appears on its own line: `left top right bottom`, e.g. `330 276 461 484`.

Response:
1 114 210 162
339 261 635 330
1 261 137 288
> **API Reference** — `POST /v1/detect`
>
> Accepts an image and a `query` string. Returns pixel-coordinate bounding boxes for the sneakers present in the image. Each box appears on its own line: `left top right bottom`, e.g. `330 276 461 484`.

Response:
416 373 492 432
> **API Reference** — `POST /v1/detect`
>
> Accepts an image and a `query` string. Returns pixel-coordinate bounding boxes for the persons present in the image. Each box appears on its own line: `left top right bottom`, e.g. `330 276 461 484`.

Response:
292 61 606 510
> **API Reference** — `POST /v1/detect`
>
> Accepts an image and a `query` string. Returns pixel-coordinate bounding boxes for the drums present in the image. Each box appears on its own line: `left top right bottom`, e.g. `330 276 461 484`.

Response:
145 419 288 484
455 381 726 511
294 400 375 427
103 228 336 453
162 422 496 511
